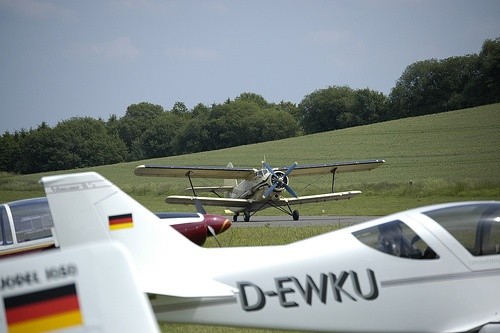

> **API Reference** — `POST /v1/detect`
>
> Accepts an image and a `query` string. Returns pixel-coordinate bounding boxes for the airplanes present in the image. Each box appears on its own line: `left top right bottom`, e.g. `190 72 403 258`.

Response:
2 170 500 333
0 196 234 267
133 160 385 219
